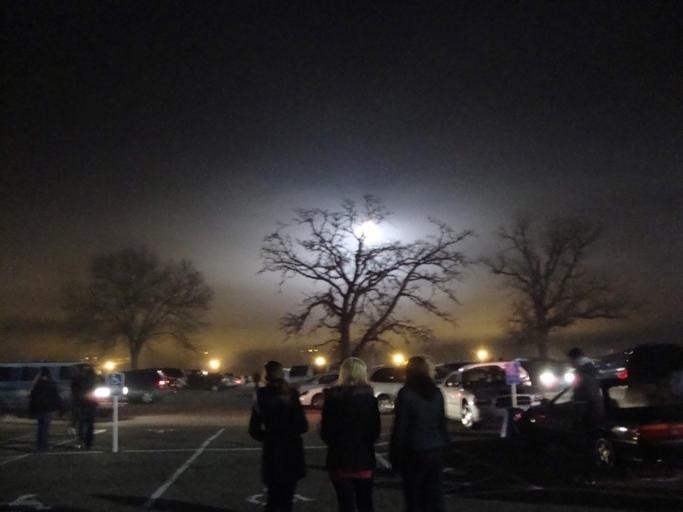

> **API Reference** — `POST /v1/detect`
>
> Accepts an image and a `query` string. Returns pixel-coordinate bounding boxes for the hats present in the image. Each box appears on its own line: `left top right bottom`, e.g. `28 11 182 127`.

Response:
566 347 583 357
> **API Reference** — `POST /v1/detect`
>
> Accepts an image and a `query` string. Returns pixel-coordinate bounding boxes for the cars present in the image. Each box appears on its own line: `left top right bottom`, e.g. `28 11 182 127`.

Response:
506 342 683 481
0 361 254 415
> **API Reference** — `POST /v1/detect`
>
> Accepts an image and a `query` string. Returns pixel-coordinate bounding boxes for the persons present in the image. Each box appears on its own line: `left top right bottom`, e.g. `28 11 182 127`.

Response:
320 357 381 512
27 367 62 451
248 361 309 511
567 347 607 432
391 355 452 512
69 361 100 449
